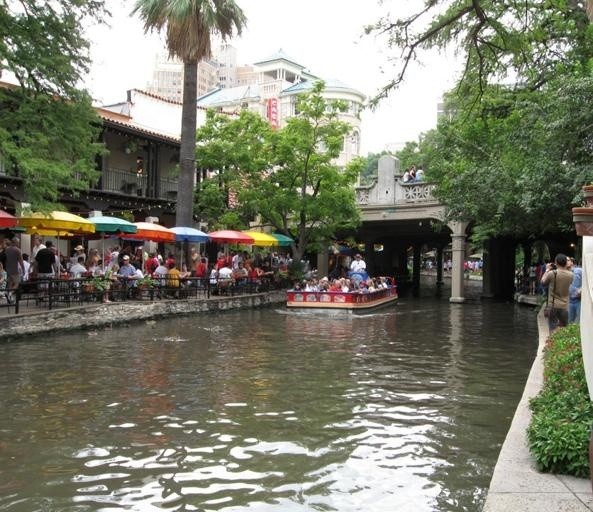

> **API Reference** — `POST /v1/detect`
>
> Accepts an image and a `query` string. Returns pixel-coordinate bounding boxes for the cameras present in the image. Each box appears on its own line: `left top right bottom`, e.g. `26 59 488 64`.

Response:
551 264 557 270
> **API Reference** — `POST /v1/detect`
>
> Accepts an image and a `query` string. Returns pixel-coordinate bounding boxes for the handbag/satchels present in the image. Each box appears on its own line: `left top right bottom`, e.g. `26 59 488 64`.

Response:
543 308 557 317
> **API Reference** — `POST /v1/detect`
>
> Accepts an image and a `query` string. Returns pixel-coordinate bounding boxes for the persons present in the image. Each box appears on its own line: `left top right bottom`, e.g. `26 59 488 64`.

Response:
0 234 208 304
402 168 408 184
209 248 292 295
293 253 389 294
409 165 415 183
415 166 424 182
419 253 582 332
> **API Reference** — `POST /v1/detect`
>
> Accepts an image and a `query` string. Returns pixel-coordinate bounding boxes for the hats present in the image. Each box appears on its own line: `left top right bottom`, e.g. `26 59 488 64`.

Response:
123 255 130 261
354 254 362 259
73 245 85 251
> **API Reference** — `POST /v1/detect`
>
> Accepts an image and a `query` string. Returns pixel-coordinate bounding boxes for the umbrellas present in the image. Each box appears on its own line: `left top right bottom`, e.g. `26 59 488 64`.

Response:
0 209 294 272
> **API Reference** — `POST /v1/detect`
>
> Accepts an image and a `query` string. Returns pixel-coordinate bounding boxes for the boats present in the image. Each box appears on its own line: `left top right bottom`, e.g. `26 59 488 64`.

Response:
284 276 399 315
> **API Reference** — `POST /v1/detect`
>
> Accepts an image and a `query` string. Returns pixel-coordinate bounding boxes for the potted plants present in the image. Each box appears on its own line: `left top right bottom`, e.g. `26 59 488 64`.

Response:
119 139 138 155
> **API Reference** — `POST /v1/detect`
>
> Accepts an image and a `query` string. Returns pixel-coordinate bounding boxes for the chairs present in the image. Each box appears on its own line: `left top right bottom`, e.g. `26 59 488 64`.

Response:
0 269 275 313
